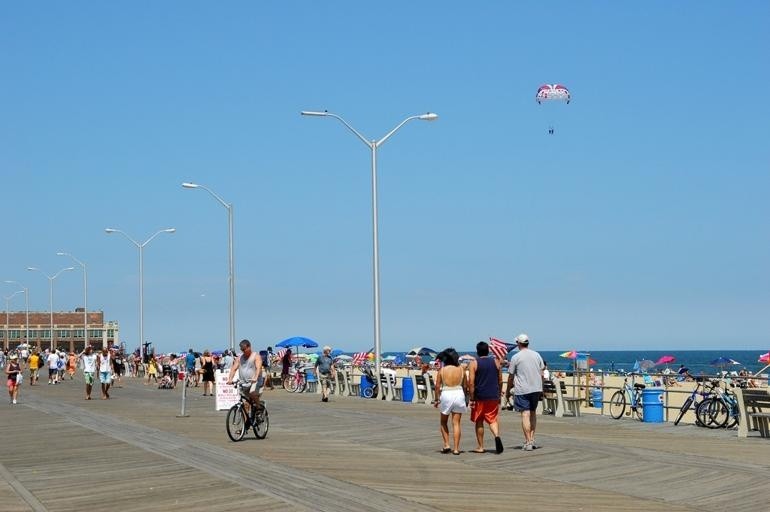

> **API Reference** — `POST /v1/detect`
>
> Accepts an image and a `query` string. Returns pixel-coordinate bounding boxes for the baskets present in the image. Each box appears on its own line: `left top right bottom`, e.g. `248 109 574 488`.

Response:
288 366 298 376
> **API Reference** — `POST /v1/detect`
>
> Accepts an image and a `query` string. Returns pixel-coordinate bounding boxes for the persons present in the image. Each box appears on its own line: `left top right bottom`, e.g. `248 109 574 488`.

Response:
659 362 760 388
503 334 547 452
313 345 336 403
226 340 263 433
0 342 298 405
434 348 469 455
542 357 551 413
414 353 424 367
469 341 505 455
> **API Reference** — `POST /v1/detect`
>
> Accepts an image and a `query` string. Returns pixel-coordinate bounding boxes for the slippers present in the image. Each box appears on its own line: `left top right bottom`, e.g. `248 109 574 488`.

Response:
440 436 504 455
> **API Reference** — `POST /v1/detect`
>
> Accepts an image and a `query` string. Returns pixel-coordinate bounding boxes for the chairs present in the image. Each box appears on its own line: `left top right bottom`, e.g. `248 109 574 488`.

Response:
640 370 675 388
722 369 769 389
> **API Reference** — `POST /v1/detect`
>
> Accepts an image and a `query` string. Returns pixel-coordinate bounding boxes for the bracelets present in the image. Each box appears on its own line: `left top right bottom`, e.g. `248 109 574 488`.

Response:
469 399 476 402
434 399 439 402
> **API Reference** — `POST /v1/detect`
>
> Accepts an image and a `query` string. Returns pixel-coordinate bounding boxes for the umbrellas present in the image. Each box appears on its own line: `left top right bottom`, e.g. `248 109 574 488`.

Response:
405 346 440 357
632 355 678 372
756 351 770 369
275 335 319 358
558 350 598 367
707 355 742 375
329 349 345 356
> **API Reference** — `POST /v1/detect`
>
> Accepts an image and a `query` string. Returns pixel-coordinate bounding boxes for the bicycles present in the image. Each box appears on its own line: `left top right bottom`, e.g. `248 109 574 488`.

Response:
225 379 271 440
282 365 307 392
610 370 647 421
674 370 761 432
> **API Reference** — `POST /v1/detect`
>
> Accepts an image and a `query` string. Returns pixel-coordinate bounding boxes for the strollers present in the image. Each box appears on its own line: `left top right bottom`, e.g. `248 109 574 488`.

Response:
360 363 396 399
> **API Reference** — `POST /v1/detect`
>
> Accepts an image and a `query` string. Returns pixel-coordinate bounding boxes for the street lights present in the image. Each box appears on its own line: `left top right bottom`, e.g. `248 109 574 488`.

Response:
2 264 76 354
103 227 176 367
297 108 438 404
55 250 90 357
181 181 238 363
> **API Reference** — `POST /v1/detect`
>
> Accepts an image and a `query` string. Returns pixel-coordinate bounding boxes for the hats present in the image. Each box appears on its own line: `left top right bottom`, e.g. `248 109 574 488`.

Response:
10 353 19 360
514 334 529 344
323 346 331 353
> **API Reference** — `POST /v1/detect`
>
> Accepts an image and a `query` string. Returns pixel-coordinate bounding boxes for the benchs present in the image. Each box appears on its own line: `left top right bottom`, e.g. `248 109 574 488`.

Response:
732 384 770 437
300 367 584 420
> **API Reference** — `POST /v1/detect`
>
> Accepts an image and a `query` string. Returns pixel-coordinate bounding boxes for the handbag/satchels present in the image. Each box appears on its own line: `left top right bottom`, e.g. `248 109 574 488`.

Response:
199 369 206 373
15 374 23 385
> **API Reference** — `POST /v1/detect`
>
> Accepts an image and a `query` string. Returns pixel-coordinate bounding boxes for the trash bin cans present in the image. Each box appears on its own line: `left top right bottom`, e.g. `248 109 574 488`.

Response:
593 390 603 407
642 389 663 422
361 374 373 397
402 378 414 402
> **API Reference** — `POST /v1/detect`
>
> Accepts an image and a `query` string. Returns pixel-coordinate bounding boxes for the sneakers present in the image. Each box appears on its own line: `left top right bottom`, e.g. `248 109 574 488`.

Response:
522 440 538 451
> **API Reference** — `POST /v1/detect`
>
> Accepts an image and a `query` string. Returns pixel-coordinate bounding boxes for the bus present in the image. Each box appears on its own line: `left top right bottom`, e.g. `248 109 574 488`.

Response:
16 343 33 358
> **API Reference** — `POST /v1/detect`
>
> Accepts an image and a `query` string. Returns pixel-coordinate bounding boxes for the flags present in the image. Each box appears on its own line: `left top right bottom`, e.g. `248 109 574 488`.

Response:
351 351 368 367
486 335 518 360
